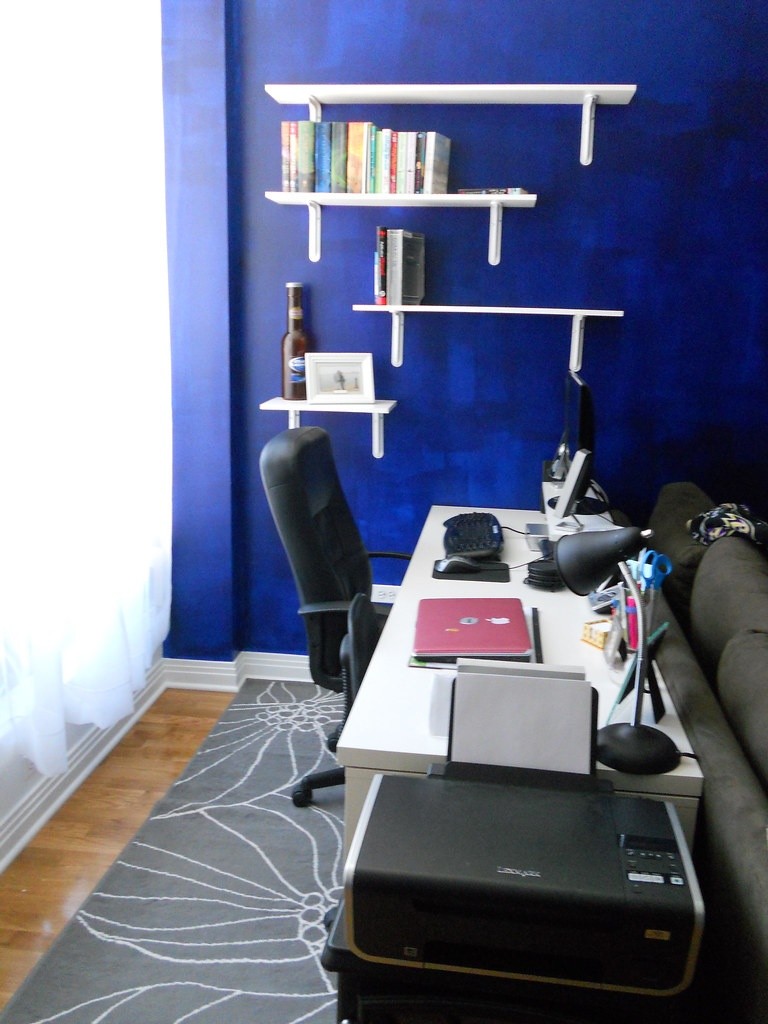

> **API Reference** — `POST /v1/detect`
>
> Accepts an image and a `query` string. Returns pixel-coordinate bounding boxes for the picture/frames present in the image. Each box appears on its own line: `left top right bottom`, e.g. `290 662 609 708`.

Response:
305 352 376 404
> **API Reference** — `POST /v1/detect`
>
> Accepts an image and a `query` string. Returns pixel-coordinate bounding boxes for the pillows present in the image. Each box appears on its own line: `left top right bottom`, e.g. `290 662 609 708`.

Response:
650 481 768 784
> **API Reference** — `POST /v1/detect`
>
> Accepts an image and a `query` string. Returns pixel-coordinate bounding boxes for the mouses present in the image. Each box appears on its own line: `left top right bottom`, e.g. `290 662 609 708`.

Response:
435 555 481 573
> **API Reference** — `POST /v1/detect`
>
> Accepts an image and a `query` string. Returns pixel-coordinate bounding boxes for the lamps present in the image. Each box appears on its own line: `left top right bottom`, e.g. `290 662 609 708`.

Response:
554 524 683 774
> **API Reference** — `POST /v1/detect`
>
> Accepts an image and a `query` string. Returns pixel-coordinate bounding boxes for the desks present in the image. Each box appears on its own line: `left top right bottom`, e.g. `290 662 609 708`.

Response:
337 505 703 867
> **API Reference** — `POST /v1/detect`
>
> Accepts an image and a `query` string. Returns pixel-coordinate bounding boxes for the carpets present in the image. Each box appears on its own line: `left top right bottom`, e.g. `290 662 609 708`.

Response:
0 676 350 1024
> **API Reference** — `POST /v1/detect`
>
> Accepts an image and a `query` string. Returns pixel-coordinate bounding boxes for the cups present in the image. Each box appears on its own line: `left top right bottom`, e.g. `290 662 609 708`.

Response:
623 595 652 652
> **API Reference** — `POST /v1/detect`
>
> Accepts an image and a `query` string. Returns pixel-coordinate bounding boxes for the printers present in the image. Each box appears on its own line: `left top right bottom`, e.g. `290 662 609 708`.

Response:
342 670 704 996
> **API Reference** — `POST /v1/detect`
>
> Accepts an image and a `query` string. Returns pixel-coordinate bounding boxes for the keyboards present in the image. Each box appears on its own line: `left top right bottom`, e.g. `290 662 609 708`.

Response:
443 512 503 558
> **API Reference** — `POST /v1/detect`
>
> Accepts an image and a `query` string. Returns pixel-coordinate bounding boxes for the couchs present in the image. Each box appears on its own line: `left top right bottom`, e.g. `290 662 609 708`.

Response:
611 508 768 1024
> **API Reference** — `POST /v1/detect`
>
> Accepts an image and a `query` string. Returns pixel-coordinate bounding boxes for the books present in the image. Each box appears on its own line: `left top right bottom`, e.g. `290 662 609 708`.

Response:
280 121 452 194
458 188 528 194
374 226 424 305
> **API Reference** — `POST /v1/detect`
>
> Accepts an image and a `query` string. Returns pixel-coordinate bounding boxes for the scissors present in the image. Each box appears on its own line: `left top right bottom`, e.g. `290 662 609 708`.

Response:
639 549 673 603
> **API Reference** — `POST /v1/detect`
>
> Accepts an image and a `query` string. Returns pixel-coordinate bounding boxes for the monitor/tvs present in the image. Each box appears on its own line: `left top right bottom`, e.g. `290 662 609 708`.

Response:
547 370 609 515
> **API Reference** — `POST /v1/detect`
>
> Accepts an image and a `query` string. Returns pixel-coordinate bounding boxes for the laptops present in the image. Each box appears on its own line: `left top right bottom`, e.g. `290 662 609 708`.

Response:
414 598 533 664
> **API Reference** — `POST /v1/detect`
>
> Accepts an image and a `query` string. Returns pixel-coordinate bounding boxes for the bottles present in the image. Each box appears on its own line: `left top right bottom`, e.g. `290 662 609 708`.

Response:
282 282 309 400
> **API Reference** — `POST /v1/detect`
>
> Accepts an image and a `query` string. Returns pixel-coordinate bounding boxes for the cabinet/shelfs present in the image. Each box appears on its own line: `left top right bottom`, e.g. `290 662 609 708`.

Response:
259 83 639 458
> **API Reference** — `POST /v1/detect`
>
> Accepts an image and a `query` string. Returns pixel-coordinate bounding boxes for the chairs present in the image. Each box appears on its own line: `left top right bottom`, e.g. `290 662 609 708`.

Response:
329 593 378 745
260 426 413 809
